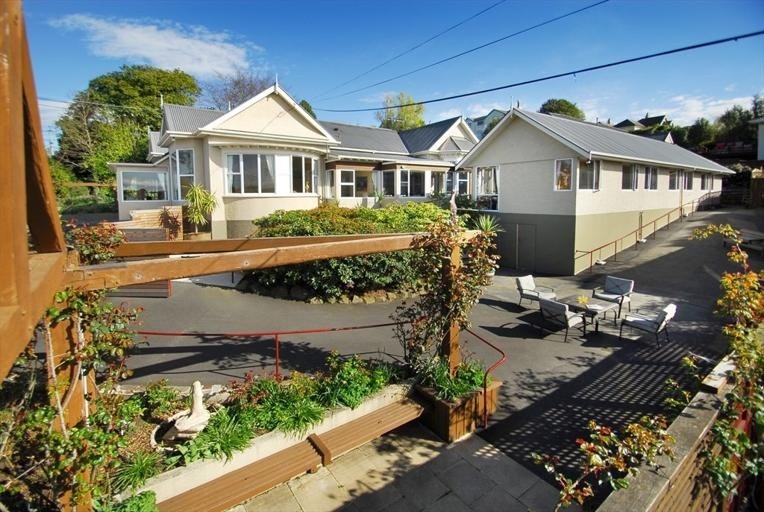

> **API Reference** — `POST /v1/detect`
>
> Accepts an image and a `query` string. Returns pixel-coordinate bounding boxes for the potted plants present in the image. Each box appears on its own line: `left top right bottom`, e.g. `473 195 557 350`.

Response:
410 352 474 446
462 357 502 418
180 183 215 243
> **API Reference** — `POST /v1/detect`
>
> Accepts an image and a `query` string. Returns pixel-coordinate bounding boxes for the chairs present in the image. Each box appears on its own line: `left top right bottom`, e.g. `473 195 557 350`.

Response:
514 273 557 308
538 296 586 343
617 302 677 349
591 274 635 318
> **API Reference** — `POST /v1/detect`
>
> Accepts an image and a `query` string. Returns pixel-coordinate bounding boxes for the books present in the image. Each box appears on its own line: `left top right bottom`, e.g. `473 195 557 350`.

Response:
586 304 603 310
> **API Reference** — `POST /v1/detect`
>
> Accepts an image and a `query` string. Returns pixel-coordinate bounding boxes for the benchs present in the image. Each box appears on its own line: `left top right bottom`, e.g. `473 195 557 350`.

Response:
137 395 427 510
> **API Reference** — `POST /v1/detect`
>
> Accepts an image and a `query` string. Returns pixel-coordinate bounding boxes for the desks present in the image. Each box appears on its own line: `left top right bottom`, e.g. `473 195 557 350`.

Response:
559 294 617 335
